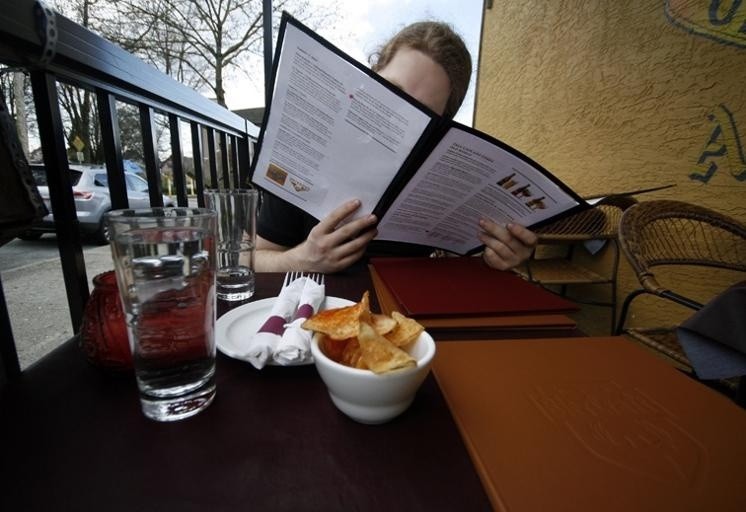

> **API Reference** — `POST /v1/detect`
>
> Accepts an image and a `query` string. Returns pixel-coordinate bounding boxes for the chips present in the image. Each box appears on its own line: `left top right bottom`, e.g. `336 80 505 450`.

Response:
300 291 425 375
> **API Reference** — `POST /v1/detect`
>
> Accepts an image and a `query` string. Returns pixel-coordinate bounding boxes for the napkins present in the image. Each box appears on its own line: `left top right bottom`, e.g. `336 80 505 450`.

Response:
676 279 744 382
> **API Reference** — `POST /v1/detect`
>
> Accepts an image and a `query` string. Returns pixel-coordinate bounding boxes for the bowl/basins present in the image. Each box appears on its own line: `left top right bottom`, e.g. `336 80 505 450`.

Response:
310 314 436 426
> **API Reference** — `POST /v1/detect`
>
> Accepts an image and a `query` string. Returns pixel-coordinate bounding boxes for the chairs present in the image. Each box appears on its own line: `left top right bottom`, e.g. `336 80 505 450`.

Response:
512 194 639 335
616 200 744 376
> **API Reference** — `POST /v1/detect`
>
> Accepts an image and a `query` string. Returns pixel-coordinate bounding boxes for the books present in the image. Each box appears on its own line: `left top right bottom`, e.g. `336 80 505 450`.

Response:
248 8 613 256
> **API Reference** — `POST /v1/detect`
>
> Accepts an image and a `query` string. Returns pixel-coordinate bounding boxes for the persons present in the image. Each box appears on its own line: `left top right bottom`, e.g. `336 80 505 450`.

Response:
236 16 539 276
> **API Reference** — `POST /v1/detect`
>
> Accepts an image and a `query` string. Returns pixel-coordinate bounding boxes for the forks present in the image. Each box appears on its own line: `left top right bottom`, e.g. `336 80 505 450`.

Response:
248 268 325 372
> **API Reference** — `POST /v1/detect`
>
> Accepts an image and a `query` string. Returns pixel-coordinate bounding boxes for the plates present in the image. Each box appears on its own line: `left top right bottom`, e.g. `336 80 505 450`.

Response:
213 295 358 362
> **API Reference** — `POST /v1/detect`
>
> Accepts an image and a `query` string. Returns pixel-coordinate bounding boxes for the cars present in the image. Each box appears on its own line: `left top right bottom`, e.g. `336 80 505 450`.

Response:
19 156 176 247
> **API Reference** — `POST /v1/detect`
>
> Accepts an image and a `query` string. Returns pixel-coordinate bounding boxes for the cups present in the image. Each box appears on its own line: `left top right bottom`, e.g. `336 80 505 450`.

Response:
101 204 218 422
202 185 259 303
